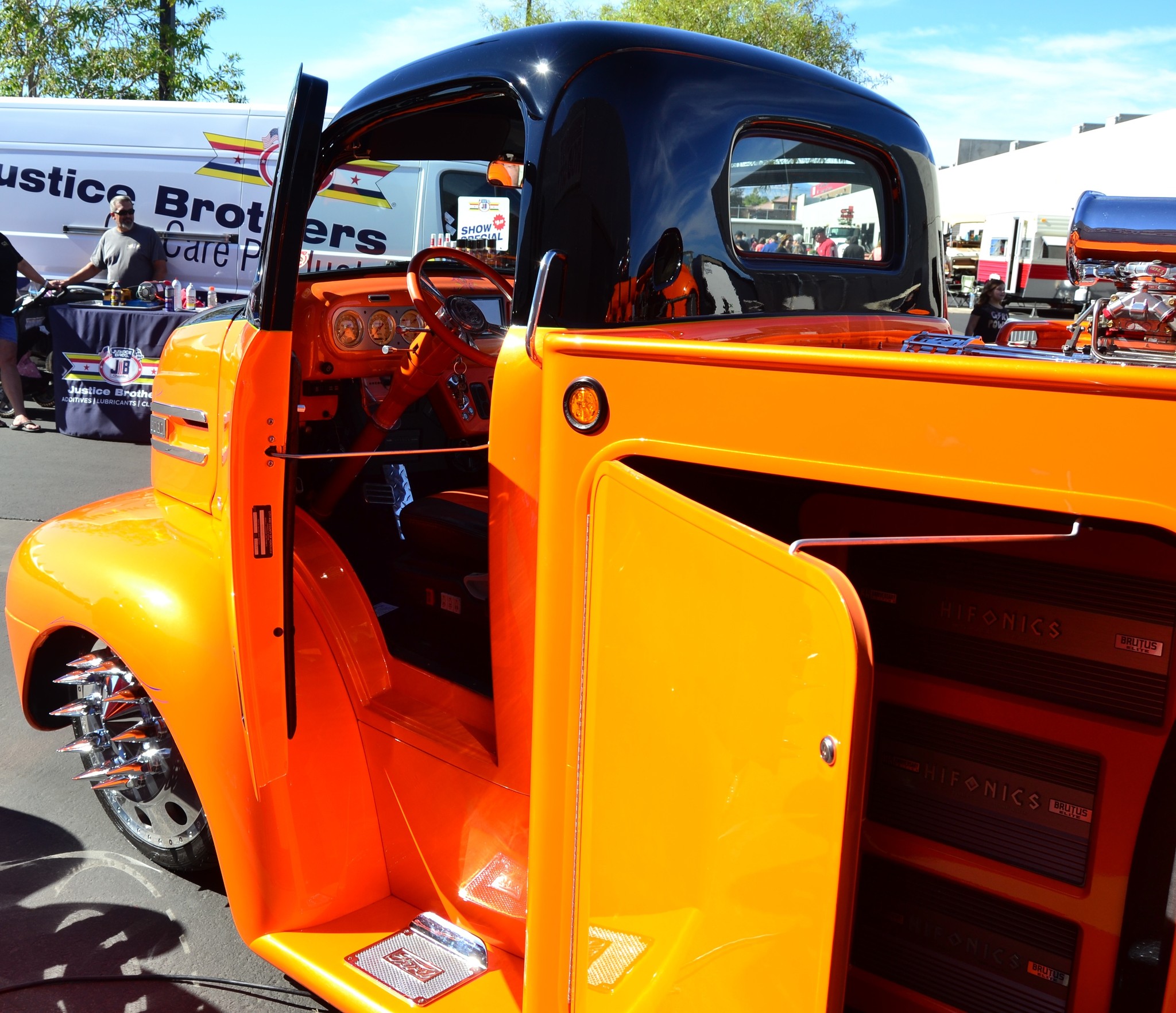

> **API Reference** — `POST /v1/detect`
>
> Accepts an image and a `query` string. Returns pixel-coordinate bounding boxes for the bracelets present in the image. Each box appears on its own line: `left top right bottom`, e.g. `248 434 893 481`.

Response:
42 278 48 287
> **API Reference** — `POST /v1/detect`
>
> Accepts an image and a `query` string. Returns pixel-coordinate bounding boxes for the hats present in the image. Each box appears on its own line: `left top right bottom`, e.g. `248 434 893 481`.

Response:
735 230 743 236
771 235 778 240
810 227 825 238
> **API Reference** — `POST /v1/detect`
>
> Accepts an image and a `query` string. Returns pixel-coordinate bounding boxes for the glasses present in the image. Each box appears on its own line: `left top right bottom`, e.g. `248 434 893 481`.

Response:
114 209 135 216
787 239 794 242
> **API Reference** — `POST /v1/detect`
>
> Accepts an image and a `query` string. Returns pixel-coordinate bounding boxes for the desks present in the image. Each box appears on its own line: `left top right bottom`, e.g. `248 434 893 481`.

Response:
49 300 213 446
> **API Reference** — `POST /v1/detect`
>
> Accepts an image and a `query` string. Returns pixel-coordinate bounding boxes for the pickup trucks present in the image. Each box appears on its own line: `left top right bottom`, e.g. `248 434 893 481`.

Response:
5 0 1176 1013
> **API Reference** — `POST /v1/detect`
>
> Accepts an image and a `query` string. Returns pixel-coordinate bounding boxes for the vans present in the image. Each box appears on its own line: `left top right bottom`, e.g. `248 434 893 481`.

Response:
0 96 523 407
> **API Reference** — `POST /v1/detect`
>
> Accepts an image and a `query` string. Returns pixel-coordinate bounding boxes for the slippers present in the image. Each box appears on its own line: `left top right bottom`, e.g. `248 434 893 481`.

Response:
10 421 41 432
0 420 6 427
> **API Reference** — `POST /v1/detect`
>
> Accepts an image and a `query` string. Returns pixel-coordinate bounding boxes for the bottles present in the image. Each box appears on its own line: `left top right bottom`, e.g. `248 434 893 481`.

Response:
207 287 218 307
172 277 184 311
165 280 175 311
185 283 196 310
111 282 121 305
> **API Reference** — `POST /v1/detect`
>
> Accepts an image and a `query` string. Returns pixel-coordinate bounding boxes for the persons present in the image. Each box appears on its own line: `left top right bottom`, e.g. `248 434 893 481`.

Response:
735 221 955 280
58 196 167 300
965 279 1010 342
0 232 61 431
